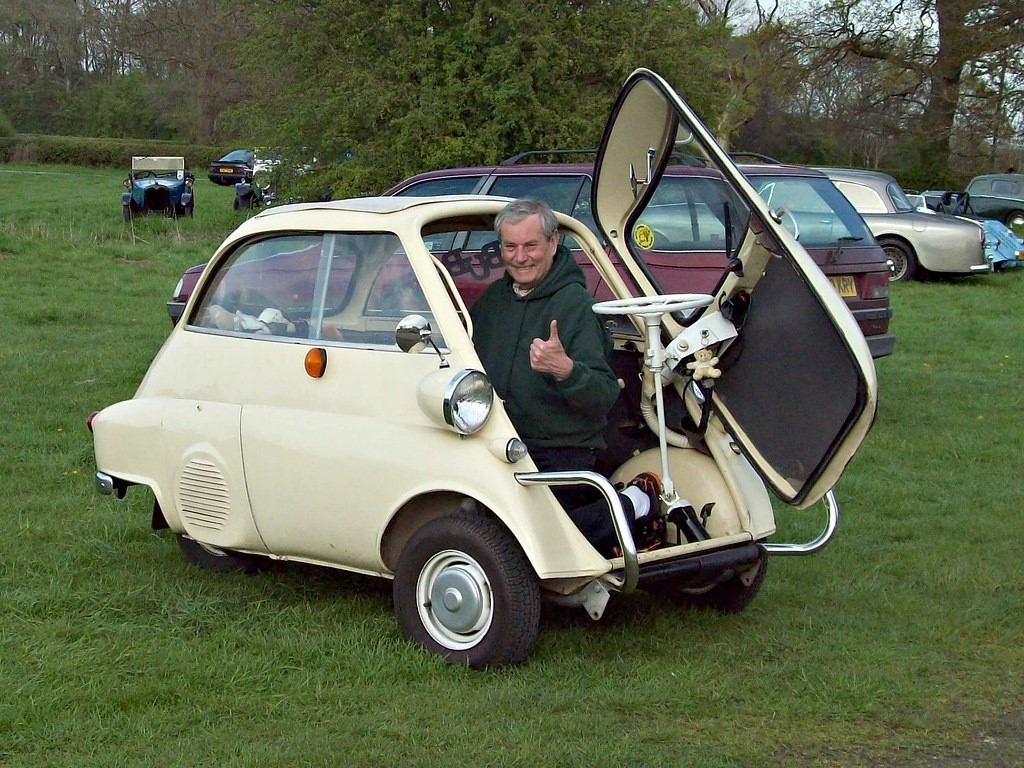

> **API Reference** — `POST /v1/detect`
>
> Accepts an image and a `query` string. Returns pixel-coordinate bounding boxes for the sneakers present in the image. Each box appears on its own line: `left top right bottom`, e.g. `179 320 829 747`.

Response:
609 471 668 557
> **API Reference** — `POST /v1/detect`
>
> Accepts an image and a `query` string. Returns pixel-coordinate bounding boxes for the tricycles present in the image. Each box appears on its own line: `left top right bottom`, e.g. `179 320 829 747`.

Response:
85 61 884 676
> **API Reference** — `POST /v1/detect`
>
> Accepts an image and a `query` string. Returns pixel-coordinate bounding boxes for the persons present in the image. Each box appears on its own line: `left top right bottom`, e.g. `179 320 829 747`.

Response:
463 197 621 511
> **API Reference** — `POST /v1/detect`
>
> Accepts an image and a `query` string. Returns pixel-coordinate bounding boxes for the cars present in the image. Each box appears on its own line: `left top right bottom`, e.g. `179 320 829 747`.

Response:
900 172 1024 271
635 161 995 284
204 147 252 185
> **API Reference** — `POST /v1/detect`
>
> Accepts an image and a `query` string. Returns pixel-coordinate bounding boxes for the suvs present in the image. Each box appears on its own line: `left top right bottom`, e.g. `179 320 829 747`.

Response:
120 153 195 224
166 150 899 422
